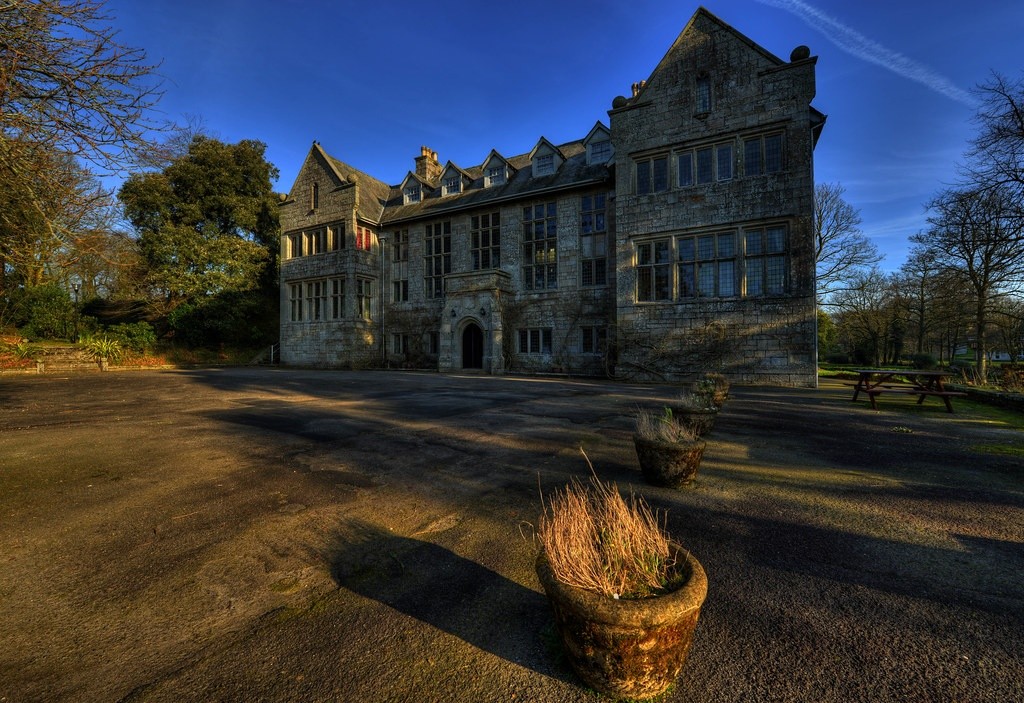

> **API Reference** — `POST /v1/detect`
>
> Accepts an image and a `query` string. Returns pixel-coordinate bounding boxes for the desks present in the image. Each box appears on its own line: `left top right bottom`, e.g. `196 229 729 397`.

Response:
852 370 957 414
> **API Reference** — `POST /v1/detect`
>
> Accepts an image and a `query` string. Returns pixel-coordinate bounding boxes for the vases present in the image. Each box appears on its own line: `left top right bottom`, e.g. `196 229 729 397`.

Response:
536 529 710 697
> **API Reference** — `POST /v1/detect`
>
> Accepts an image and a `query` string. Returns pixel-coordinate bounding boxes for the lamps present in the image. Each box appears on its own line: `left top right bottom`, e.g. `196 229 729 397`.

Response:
451 308 456 317
480 306 486 316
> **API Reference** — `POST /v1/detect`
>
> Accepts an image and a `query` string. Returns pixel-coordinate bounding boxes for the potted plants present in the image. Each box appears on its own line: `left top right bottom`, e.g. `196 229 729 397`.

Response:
631 405 706 490
681 372 730 437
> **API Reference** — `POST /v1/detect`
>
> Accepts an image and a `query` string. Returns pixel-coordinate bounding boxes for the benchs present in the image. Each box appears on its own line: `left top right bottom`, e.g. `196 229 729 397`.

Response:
842 382 968 400
